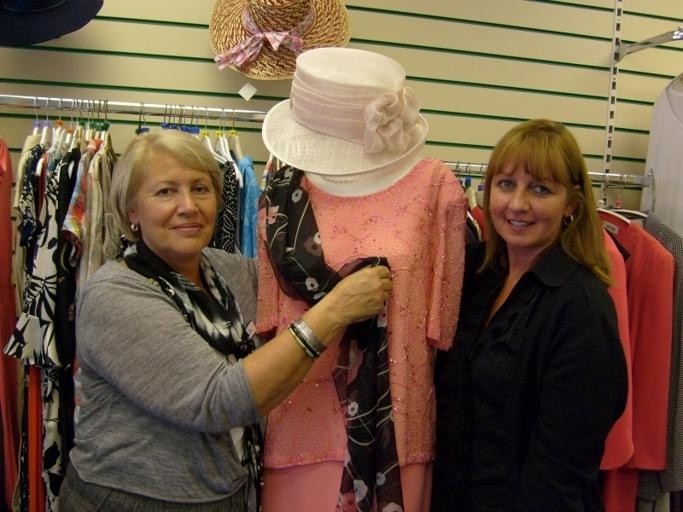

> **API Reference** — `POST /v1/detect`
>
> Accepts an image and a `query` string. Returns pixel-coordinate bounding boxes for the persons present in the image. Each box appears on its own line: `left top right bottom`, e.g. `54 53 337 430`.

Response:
419 116 630 512
50 126 397 512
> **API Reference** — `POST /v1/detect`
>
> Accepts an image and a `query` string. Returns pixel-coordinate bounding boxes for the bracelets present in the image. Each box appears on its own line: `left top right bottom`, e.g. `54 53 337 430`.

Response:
286 318 328 361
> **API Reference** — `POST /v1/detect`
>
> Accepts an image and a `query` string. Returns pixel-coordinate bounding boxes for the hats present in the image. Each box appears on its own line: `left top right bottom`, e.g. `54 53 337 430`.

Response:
261 46 430 177
208 0 352 81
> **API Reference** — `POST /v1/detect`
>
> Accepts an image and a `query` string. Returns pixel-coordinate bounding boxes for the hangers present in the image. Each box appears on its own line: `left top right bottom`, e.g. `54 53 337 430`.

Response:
30 96 246 188
453 161 647 242
